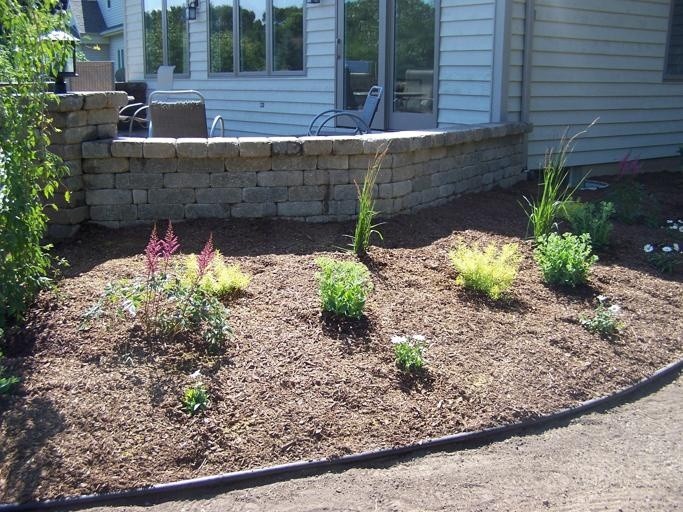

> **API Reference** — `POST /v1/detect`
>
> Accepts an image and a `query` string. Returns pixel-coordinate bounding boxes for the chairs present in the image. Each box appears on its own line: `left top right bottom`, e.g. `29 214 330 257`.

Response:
119 65 175 134
349 73 371 108
307 85 383 135
404 69 433 113
147 89 224 137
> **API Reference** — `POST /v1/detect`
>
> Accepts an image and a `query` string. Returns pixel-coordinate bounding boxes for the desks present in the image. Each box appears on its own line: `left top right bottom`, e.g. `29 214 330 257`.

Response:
353 90 424 102
